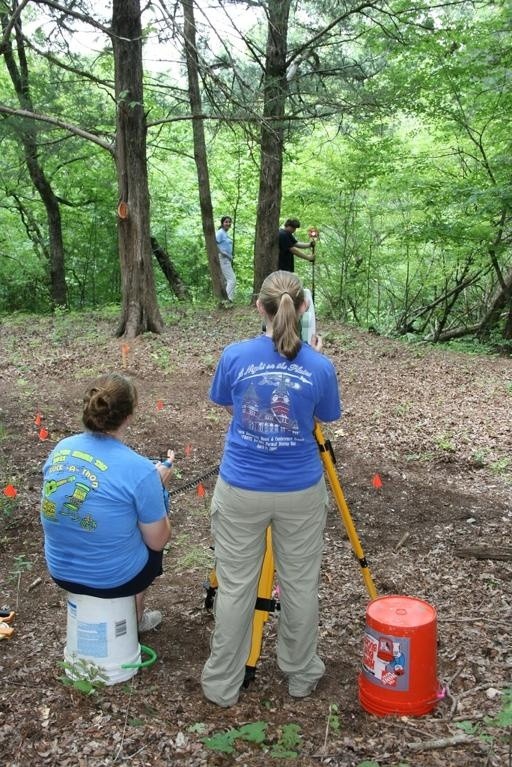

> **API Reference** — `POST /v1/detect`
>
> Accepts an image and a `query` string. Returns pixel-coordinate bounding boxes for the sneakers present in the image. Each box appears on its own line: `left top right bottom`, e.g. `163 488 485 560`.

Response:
138 610 161 633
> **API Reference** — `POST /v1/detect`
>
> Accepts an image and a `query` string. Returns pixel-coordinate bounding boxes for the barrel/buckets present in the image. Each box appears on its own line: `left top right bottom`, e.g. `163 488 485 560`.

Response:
63 590 157 686
357 596 439 716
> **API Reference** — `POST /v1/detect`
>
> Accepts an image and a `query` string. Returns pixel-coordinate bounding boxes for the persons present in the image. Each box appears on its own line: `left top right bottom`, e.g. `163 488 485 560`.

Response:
36 370 176 636
197 267 343 711
276 216 318 272
215 216 239 303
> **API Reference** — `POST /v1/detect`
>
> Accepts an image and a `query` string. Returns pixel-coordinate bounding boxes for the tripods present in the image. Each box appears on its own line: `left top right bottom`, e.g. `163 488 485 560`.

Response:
204 421 381 688
